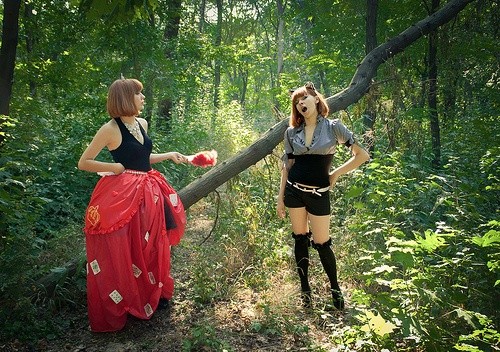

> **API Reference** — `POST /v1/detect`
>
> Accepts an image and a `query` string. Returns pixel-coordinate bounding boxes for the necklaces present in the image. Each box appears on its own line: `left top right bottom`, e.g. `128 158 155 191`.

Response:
121 118 142 139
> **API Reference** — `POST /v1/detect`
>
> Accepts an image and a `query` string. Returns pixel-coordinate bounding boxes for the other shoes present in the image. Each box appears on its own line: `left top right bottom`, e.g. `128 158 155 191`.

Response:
158 298 168 309
301 287 312 307
331 283 344 310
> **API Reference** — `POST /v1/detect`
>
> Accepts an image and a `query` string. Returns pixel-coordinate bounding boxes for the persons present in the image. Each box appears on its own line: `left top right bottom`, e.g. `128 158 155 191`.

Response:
78 79 188 333
277 81 369 314
